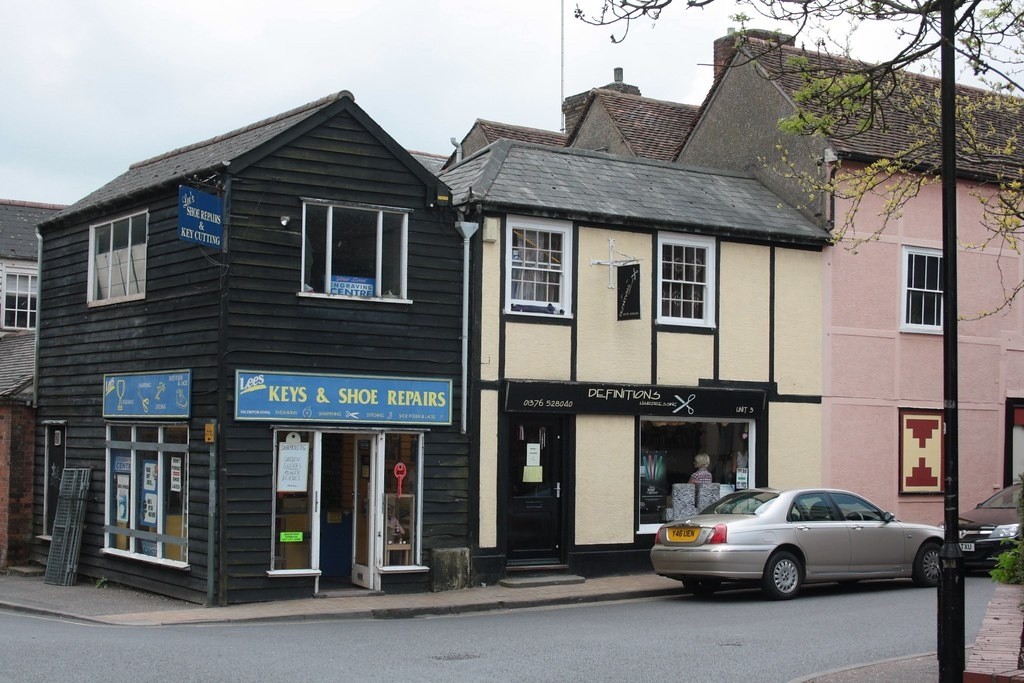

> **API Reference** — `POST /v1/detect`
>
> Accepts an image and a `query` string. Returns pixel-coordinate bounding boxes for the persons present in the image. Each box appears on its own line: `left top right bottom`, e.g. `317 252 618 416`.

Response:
687 454 713 483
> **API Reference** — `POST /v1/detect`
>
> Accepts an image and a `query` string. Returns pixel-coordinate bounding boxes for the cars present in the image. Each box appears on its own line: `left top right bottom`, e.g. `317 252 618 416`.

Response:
939 482 1024 577
650 487 945 601
